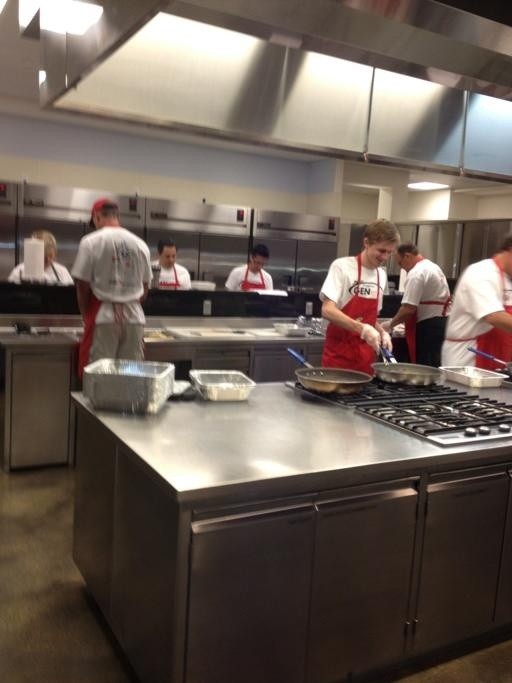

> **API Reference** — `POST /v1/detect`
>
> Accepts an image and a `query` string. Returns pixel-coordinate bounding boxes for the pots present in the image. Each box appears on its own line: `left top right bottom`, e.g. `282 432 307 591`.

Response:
372 343 445 386
465 344 512 373
283 344 375 394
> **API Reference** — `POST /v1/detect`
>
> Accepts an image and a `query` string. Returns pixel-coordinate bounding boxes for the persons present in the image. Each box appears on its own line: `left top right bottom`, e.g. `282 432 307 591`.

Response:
225 243 275 292
6 228 74 289
441 230 512 370
318 217 401 374
150 237 190 290
72 196 153 381
380 241 451 365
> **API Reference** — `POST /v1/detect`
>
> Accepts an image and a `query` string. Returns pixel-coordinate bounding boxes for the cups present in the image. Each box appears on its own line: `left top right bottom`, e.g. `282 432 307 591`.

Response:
150 263 161 290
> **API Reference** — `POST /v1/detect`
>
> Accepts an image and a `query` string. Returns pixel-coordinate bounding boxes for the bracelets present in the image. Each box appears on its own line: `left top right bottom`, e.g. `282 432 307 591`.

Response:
360 321 371 340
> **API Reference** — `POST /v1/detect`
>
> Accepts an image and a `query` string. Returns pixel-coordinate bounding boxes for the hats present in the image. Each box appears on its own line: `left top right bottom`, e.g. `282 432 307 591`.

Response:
88 199 118 229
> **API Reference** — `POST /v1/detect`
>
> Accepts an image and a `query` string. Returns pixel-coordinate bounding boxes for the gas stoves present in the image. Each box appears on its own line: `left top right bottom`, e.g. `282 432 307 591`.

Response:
285 372 512 450
